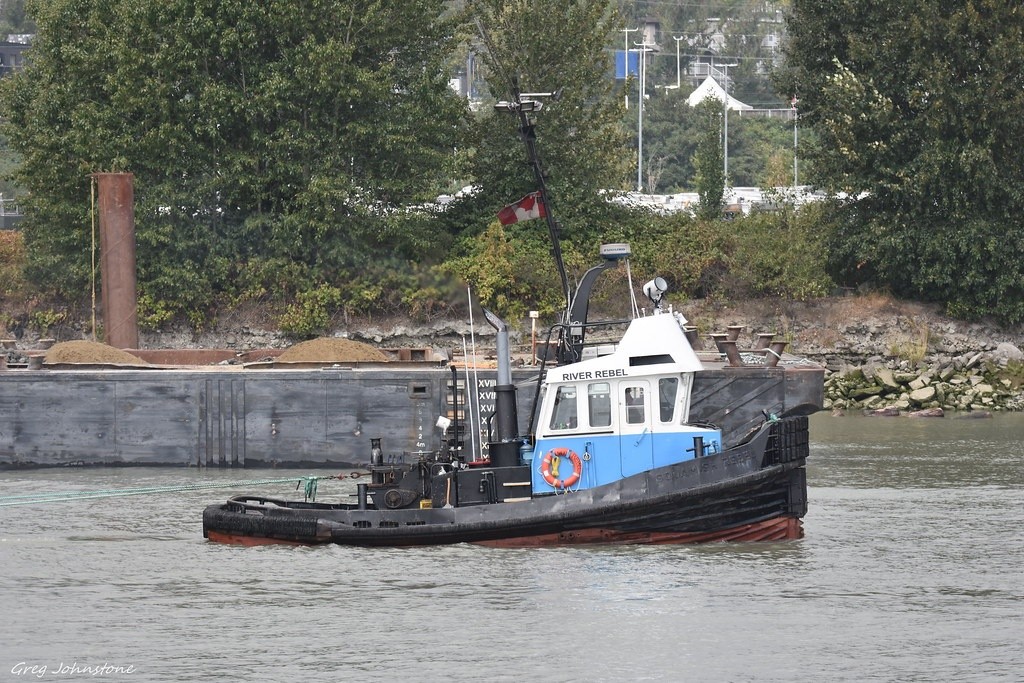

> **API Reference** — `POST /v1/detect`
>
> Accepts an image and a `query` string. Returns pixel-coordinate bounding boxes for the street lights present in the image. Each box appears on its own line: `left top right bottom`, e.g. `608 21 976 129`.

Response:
618 26 639 109
628 47 656 193
714 62 739 188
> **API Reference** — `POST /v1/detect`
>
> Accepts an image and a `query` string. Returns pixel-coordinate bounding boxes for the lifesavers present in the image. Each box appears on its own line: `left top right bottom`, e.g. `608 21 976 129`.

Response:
540 448 581 488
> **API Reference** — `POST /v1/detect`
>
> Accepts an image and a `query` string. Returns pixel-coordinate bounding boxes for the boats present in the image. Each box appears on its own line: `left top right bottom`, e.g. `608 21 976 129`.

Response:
202 72 824 550
